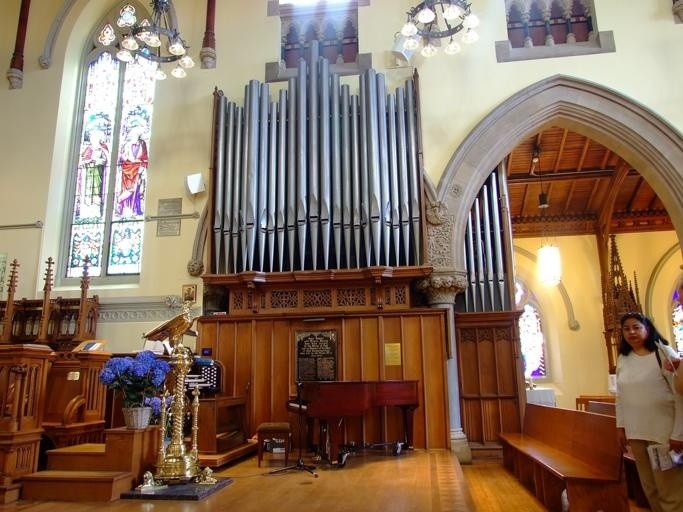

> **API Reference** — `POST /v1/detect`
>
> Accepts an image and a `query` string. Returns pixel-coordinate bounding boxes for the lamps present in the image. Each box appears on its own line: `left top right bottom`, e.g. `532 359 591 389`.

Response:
533 144 549 209
401 0 479 58
116 0 195 81
537 210 562 287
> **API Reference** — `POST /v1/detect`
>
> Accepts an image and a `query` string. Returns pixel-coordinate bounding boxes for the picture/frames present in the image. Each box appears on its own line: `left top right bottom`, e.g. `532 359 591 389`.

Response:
182 284 197 303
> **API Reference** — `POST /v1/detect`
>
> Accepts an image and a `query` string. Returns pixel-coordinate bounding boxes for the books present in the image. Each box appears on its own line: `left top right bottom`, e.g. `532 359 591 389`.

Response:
143 319 170 336
653 339 678 377
645 443 682 472
72 339 109 351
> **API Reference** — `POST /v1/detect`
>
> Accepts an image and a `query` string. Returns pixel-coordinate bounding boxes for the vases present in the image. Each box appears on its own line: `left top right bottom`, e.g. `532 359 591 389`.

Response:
121 405 154 430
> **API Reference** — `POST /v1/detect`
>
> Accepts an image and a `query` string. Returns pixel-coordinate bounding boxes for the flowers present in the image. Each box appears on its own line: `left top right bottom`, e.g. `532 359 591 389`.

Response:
98 350 172 410
143 398 174 440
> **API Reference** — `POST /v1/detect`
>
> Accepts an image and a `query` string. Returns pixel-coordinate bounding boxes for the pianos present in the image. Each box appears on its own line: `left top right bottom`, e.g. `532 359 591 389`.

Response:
284 380 418 468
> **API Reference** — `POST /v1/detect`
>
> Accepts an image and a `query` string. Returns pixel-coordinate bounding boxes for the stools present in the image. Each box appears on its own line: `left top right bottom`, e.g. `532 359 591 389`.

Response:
257 422 291 468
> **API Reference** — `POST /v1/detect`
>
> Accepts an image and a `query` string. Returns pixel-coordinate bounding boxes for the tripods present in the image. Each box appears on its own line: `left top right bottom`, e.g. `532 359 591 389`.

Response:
267 379 319 478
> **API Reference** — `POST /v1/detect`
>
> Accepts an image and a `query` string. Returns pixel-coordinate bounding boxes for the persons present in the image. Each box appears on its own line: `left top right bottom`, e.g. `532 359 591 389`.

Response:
663 356 680 373
614 311 682 512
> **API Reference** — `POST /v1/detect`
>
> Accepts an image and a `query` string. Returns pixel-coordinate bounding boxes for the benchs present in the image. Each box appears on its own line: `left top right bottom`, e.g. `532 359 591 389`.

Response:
497 403 629 512
588 399 650 512
